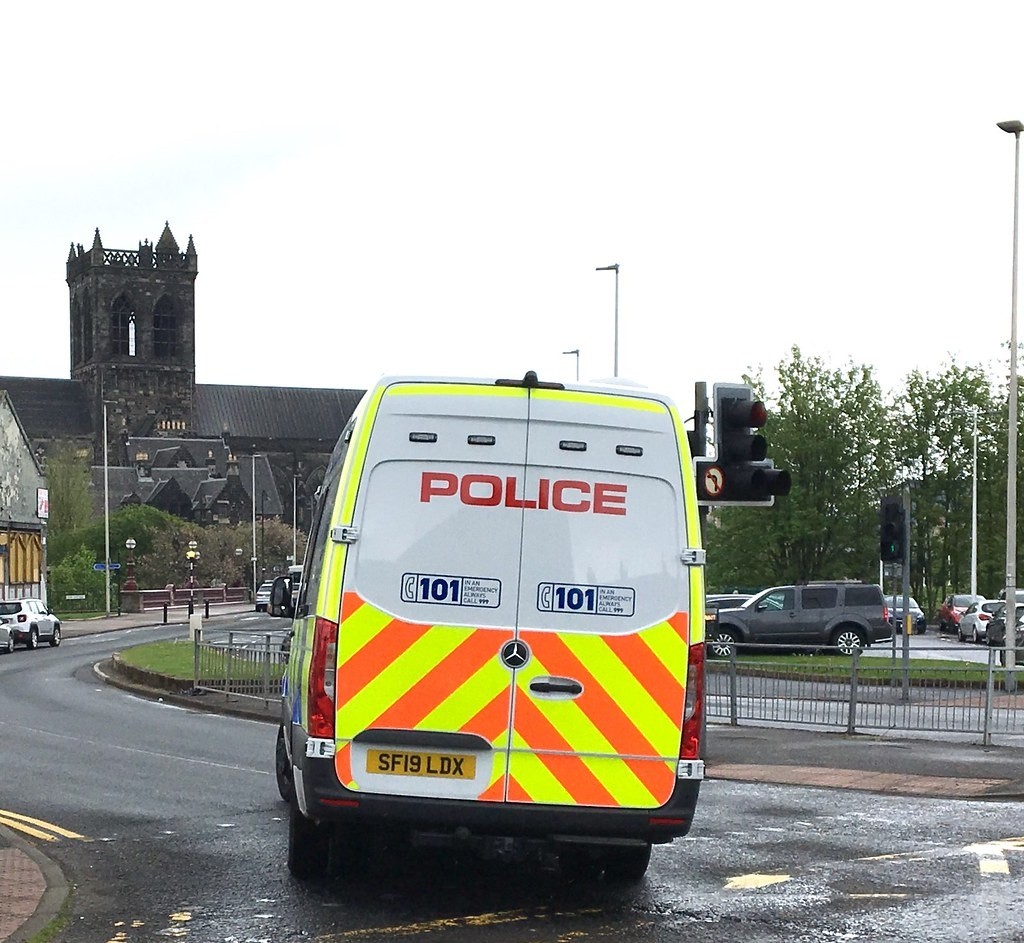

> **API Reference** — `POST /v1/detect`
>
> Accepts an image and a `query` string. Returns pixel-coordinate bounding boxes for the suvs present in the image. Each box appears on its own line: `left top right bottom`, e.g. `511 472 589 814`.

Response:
0 597 62 649
706 582 894 657
998 588 1024 603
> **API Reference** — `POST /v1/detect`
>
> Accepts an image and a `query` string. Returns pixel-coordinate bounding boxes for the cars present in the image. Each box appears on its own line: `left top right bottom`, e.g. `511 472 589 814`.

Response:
939 595 986 635
957 599 1005 644
987 604 1024 645
706 593 785 614
255 584 276 611
884 594 926 634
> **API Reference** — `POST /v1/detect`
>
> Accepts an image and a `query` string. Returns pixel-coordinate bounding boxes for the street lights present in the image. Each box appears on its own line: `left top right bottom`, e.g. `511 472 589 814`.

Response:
562 349 580 382
186 539 201 588
123 537 139 590
252 454 267 601
946 404 1002 596
596 264 618 379
292 474 302 566
102 400 119 613
994 119 1024 691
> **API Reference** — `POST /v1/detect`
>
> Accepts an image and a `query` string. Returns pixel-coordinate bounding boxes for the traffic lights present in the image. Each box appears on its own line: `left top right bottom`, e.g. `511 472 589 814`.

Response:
692 454 793 509
880 495 906 564
713 383 768 499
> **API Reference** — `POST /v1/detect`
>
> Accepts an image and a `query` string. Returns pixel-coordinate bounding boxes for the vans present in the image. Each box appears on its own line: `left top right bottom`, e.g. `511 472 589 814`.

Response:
266 371 711 897
